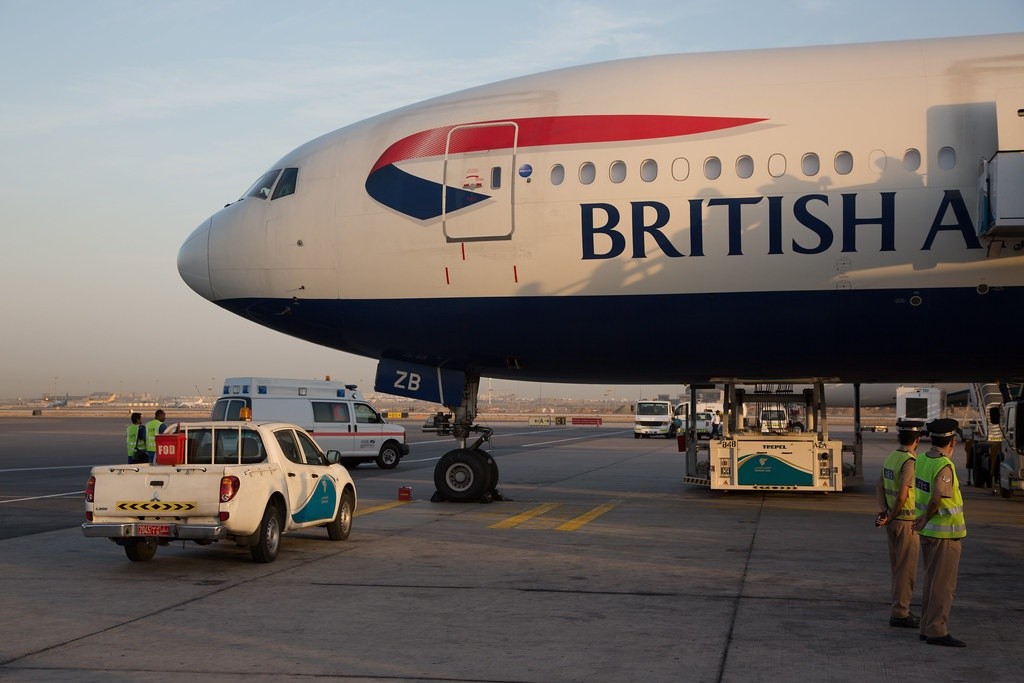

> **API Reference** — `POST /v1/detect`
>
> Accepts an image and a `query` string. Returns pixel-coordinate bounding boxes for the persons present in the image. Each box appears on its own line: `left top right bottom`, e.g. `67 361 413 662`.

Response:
144 409 168 464
709 410 721 439
910 418 970 647
873 420 924 630
125 413 146 465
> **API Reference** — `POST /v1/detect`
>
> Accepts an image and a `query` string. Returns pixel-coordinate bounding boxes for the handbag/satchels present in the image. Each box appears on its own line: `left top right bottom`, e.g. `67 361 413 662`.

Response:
132 448 149 464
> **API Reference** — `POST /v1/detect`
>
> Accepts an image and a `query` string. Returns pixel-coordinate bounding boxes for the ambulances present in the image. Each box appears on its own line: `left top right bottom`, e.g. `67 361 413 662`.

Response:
211 376 407 469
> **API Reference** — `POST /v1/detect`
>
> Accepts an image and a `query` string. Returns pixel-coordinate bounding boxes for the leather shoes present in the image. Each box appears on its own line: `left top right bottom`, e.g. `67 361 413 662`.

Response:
889 612 921 629
919 634 966 648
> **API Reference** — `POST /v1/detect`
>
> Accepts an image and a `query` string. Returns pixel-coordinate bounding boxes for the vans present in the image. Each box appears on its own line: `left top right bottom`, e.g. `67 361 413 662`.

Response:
895 385 945 437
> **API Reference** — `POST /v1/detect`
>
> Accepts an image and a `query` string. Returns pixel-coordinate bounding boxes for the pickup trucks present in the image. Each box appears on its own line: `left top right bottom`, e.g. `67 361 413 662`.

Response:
630 399 741 439
83 417 355 563
760 405 789 436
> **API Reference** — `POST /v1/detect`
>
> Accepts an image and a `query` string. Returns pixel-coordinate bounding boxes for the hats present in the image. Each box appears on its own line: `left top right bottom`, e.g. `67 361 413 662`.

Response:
927 418 959 437
896 421 924 433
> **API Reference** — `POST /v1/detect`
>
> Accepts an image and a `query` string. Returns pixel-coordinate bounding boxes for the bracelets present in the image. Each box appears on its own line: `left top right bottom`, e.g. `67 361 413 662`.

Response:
885 508 889 513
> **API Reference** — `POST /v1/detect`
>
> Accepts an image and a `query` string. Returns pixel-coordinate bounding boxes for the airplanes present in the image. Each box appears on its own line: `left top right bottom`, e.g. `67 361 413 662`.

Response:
177 35 1024 506
46 393 115 407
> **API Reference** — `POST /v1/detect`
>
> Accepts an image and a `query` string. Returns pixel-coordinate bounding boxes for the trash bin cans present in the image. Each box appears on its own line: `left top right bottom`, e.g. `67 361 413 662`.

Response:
154 433 185 465
677 432 686 452
